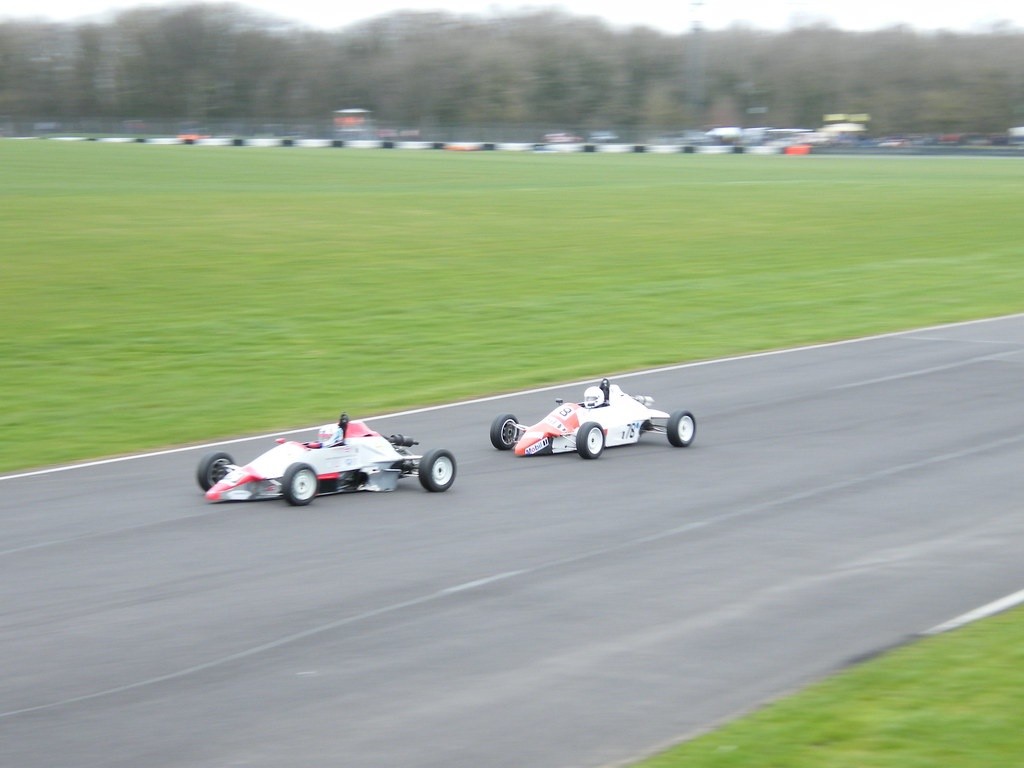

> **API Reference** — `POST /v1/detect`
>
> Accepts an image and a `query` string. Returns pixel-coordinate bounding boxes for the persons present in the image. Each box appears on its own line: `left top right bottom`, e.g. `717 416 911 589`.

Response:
318 423 344 447
584 386 606 409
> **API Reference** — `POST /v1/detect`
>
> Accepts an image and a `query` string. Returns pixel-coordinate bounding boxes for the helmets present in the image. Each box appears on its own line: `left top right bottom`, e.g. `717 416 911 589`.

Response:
584 386 605 408
319 423 344 448
